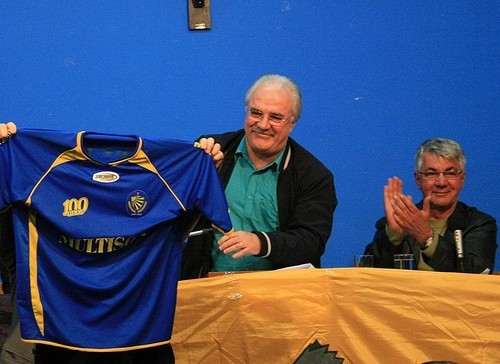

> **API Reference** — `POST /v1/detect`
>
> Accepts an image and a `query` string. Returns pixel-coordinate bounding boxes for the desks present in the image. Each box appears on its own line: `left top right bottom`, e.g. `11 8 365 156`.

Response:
170 267 500 364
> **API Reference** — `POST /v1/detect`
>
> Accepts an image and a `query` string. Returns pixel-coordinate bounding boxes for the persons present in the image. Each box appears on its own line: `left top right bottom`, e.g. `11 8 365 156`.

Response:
360 138 498 274
0 122 36 364
179 74 338 279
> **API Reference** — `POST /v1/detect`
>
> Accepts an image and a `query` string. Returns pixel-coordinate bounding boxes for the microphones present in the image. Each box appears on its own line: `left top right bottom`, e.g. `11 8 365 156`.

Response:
446 214 466 273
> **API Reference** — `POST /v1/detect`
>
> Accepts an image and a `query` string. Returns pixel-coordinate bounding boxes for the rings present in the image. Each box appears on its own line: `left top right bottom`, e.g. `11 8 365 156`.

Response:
239 242 243 249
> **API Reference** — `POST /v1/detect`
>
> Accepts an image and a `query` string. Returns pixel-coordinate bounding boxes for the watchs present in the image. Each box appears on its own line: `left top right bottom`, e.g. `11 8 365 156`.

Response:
419 227 435 251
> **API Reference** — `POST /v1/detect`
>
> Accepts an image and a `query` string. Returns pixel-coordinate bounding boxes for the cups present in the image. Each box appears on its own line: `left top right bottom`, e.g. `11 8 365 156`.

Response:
355 255 373 267
394 254 415 270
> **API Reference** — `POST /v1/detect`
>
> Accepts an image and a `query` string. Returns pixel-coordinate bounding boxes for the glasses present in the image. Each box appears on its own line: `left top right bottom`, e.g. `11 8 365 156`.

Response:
245 105 295 123
414 167 462 178
